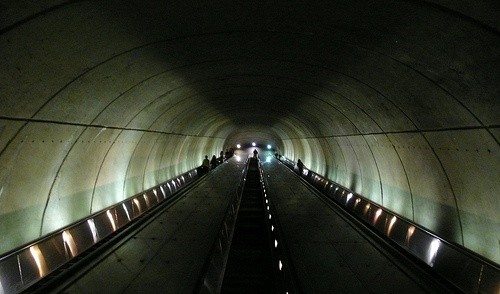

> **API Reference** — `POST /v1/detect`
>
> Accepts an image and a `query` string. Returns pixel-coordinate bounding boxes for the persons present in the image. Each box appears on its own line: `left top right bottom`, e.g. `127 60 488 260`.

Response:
253 148 258 159
201 147 234 175
275 153 282 161
297 159 304 176
